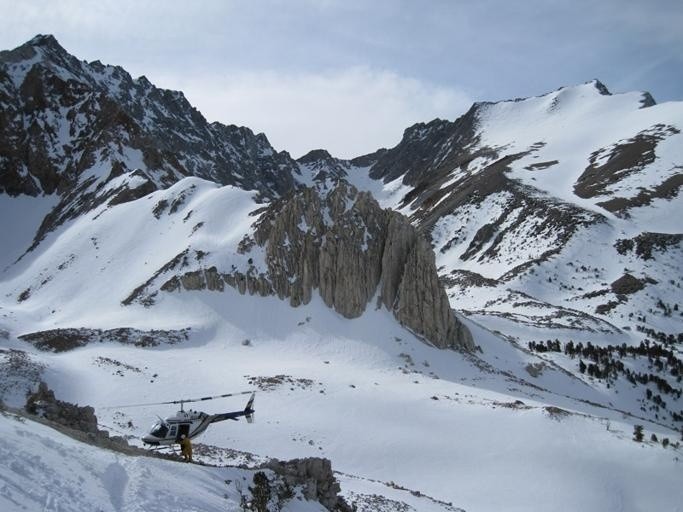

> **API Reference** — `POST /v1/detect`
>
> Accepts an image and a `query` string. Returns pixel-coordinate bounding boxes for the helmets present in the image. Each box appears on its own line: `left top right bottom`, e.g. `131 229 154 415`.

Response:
181 434 186 439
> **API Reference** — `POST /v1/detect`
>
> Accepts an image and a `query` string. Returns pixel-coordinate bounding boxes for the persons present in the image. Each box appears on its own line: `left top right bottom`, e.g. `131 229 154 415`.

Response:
178 434 193 462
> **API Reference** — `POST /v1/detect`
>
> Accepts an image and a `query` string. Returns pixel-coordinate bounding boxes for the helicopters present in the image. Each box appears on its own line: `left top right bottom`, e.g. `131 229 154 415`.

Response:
99 389 256 456
